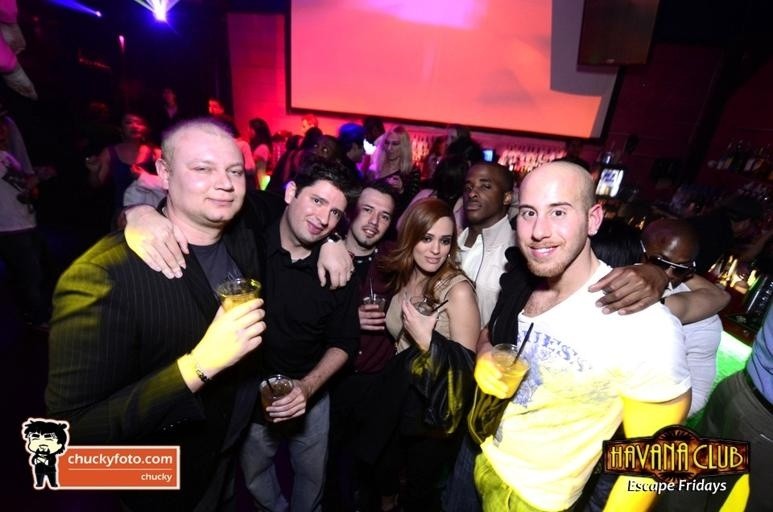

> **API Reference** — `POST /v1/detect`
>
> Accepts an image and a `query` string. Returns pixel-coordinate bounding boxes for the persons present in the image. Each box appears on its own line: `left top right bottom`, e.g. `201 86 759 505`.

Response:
2 86 773 512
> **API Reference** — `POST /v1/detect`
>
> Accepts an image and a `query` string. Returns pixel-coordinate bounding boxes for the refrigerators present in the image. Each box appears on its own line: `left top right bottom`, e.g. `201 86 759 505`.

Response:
588 162 626 208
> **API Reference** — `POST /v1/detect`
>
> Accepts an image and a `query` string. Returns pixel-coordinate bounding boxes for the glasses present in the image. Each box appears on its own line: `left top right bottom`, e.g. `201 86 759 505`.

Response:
638 239 696 279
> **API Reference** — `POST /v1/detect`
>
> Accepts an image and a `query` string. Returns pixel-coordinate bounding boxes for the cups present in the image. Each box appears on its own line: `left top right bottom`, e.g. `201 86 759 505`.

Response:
490 343 531 400
258 373 294 423
403 294 437 340
362 296 384 314
217 277 261 311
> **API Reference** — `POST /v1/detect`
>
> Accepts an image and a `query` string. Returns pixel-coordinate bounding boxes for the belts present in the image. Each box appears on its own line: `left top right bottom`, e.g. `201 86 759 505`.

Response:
742 366 773 414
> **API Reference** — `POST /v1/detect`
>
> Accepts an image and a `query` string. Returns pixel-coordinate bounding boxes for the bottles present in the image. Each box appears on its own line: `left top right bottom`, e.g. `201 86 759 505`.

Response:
501 142 568 175
602 141 617 167
411 136 431 156
714 139 768 173
707 254 756 296
736 181 769 202
